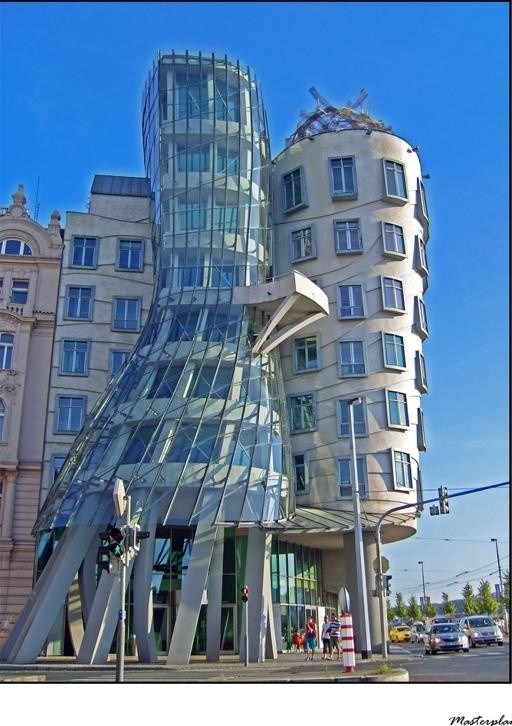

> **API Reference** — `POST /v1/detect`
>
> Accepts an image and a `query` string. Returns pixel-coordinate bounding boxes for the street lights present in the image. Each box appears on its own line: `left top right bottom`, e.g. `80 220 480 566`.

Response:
345 395 375 659
418 561 425 603
489 538 503 597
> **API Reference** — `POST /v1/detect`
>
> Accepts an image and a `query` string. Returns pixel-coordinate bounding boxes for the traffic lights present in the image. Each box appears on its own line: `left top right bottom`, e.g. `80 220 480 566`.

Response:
109 527 125 558
241 585 249 603
97 531 111 574
132 523 150 552
383 575 393 597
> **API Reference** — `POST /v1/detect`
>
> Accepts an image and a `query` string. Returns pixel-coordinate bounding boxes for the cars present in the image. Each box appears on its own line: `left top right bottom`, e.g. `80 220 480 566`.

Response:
389 615 503 655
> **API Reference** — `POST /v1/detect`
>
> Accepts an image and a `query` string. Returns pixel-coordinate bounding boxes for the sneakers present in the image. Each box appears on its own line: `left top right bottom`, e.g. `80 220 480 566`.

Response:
306 654 341 661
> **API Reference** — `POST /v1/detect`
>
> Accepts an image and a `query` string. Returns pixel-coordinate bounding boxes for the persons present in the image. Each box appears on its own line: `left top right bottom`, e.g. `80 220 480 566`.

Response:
326 612 342 661
321 614 333 659
291 615 316 663
